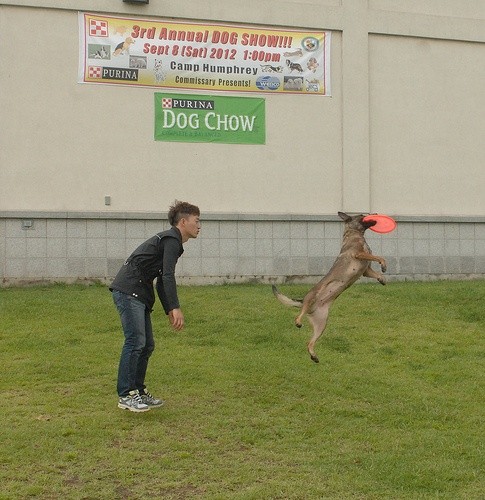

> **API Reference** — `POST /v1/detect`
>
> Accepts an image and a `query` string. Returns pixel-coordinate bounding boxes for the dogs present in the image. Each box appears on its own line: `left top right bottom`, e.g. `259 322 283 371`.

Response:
272 211 387 364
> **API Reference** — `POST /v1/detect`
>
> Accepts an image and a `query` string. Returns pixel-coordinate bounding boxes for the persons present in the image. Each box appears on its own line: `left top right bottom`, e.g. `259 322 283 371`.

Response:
107 198 201 414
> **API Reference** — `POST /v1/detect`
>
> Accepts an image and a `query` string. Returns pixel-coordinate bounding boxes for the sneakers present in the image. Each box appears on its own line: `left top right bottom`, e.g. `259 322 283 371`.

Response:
140 388 163 408
117 389 151 413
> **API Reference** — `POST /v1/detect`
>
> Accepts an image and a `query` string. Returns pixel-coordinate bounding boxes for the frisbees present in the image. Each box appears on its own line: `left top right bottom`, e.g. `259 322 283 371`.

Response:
362 214 397 234
